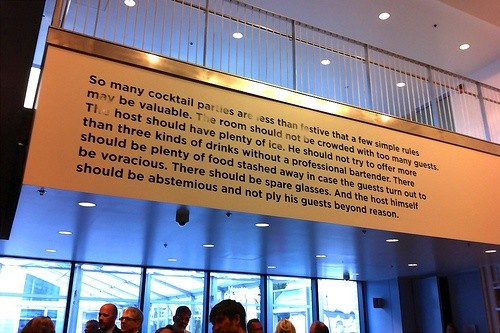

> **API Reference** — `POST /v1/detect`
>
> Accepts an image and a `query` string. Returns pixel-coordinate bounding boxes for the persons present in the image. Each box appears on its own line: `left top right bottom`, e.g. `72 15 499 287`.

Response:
98 304 122 333
20 316 55 333
274 320 296 333
247 319 263 333
84 320 100 333
310 322 329 333
119 307 144 333
155 306 192 333
210 299 246 333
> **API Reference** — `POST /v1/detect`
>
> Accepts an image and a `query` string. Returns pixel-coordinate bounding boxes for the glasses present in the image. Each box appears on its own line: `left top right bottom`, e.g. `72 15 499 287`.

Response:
98 313 109 317
121 317 137 321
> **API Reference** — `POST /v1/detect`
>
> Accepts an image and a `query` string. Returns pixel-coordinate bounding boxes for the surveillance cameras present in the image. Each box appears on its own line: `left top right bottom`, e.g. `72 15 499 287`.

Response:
176 209 189 226
343 274 349 280
